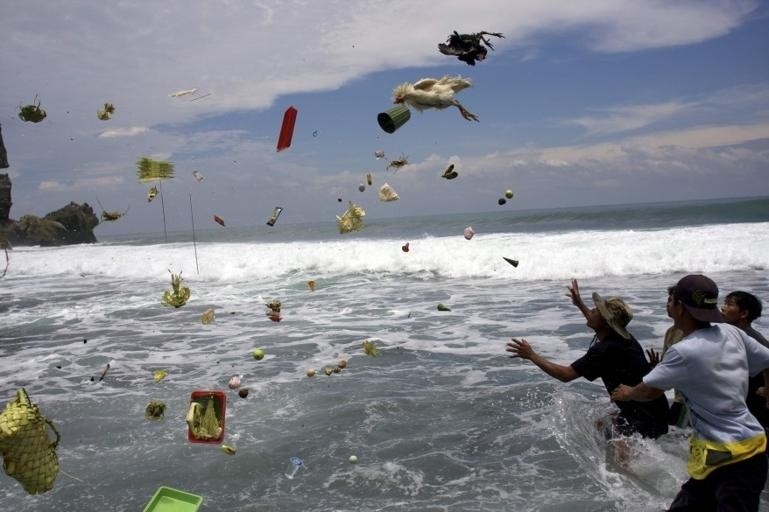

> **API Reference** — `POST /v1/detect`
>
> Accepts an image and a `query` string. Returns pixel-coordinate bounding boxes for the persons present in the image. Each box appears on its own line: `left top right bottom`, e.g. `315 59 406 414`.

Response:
503 272 670 468
610 274 768 512
721 288 768 426
644 284 698 433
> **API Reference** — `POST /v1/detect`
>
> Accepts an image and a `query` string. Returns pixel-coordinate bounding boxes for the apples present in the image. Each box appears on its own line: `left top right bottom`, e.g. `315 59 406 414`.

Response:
506 190 513 198
253 350 265 360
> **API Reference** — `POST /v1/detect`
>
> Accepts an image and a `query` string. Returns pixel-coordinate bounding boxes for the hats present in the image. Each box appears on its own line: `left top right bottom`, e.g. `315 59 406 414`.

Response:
591 291 633 340
675 274 724 324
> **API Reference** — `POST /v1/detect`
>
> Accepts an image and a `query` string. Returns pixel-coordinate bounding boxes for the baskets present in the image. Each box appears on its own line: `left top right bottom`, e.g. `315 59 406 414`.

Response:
0 387 61 497
